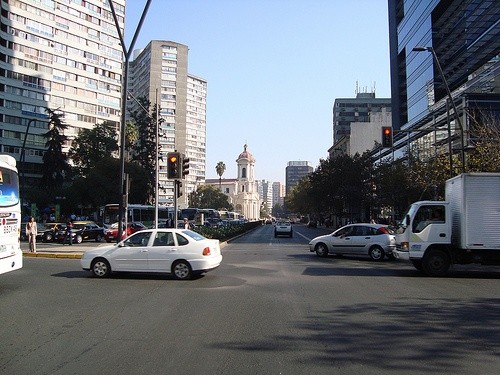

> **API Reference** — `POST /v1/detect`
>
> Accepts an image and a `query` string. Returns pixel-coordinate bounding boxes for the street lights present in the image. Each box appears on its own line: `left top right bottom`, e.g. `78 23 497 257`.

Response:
412 47 477 173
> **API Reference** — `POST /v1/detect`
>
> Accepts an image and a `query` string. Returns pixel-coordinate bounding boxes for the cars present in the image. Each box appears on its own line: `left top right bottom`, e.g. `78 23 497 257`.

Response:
273 220 293 238
309 223 396 262
80 228 223 279
21 222 105 244
103 222 148 243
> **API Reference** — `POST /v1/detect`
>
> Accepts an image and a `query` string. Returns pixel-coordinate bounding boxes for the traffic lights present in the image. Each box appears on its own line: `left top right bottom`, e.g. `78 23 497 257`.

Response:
381 126 393 148
166 150 189 198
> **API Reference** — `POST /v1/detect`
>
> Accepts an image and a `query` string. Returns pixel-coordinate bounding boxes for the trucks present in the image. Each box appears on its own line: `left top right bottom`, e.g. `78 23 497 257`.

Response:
392 171 500 276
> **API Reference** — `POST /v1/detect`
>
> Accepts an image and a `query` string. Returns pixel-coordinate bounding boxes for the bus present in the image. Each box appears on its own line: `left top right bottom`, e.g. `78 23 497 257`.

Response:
0 154 23 275
176 208 249 228
101 203 176 229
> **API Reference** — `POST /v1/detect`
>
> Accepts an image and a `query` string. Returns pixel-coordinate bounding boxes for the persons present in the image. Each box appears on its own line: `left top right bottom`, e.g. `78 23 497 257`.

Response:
426 209 442 220
166 217 174 227
370 217 397 226
63 217 72 246
183 217 190 229
340 227 352 239
68 212 90 222
26 216 37 253
42 214 47 223
